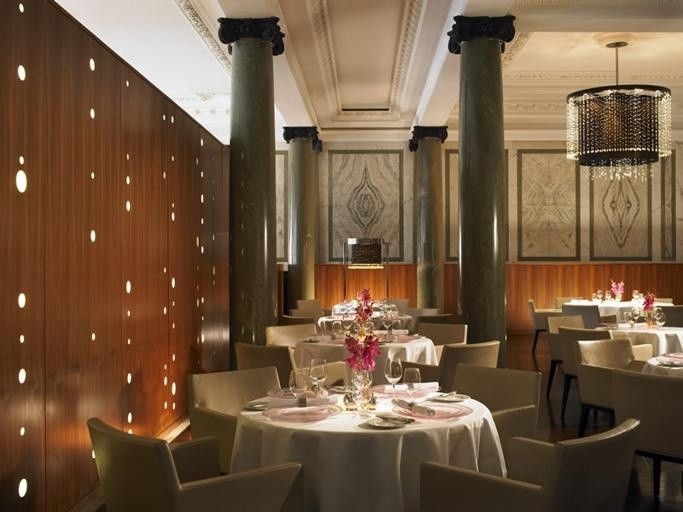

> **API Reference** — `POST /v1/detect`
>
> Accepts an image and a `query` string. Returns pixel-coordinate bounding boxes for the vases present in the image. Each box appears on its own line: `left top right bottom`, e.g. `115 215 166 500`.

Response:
344 365 376 410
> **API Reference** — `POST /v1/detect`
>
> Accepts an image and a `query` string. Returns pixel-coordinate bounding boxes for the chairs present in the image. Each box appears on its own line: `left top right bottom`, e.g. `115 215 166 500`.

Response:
236 343 350 388
187 367 281 474
280 299 452 335
452 363 542 471
528 298 618 356
397 340 500 384
419 418 641 512
265 323 317 354
88 417 301 512
417 322 468 366
581 363 683 496
547 314 652 438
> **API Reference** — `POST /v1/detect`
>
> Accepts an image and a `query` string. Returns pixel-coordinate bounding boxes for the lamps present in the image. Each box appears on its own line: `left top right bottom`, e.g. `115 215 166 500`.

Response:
566 33 672 181
342 237 390 300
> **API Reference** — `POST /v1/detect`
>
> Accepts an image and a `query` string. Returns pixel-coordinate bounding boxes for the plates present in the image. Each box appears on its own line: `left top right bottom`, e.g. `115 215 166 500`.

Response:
262 406 332 422
370 385 442 394
392 402 472 422
267 388 298 401
658 361 682 367
366 417 414 428
240 402 269 410
430 394 470 402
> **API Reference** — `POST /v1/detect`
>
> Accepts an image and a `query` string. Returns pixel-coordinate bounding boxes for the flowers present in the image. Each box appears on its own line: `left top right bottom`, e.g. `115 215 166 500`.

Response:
610 278 625 295
355 288 373 324
345 334 382 371
642 293 654 311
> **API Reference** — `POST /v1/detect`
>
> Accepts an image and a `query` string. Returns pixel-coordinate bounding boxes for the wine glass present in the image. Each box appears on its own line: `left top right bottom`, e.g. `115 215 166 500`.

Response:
591 290 643 305
331 292 400 342
623 307 665 328
289 358 421 418
